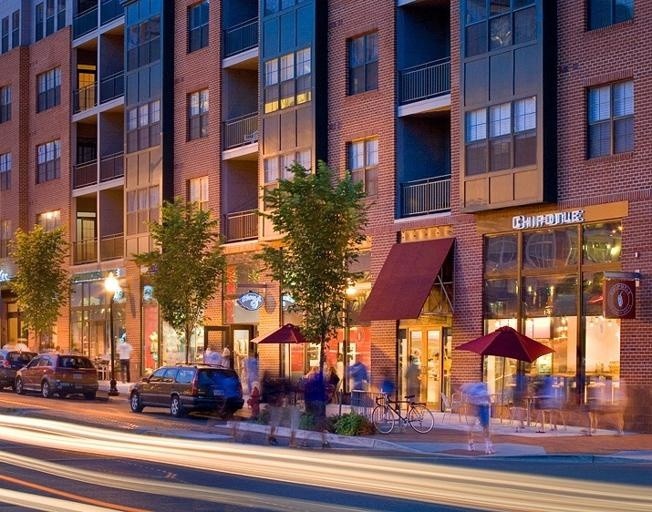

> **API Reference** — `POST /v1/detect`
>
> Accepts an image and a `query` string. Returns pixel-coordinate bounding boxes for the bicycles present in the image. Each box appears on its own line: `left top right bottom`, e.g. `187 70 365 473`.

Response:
372 394 434 434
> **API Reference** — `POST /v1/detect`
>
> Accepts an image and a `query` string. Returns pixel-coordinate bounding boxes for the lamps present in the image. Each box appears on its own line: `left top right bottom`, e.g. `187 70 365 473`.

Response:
550 315 622 344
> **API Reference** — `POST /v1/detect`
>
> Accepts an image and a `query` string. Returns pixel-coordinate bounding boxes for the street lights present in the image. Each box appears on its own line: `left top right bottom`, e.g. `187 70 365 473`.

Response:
104 271 120 394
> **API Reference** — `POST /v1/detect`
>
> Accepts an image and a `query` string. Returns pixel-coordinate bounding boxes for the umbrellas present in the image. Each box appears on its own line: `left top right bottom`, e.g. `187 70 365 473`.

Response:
248 322 323 383
455 326 555 415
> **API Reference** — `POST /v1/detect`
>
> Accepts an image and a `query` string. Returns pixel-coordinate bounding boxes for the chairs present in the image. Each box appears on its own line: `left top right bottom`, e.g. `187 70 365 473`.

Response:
437 392 551 424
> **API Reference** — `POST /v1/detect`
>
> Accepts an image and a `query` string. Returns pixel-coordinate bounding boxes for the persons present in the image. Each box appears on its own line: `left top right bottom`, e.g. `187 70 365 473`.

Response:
200 345 426 451
451 367 629 455
116 337 135 384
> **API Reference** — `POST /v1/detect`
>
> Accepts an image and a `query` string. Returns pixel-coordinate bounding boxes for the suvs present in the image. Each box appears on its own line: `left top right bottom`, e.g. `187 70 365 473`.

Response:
128 363 244 419
1 350 98 401
